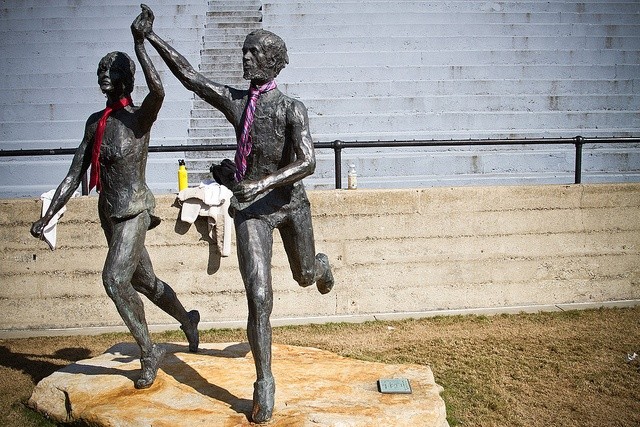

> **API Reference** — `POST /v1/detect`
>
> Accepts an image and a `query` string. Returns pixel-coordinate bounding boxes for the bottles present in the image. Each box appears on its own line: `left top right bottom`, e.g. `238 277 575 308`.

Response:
348 164 358 190
178 159 188 191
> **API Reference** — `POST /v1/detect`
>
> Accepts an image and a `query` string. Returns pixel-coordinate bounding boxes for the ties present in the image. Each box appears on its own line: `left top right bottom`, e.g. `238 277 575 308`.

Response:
233 80 277 179
87 99 131 194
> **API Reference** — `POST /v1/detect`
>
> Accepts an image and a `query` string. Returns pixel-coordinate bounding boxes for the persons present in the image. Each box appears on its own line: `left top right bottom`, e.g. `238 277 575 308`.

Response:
29 11 200 390
140 4 335 424
